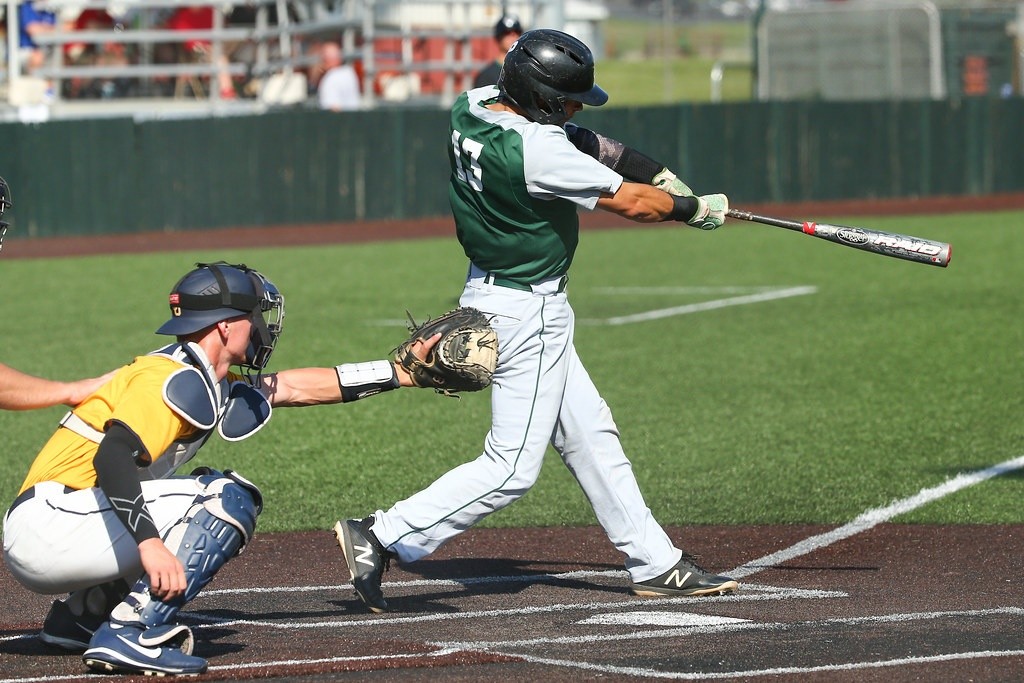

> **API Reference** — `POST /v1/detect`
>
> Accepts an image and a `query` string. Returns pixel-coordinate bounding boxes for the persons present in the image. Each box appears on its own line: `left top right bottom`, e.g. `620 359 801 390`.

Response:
3 260 499 677
475 18 522 88
16 0 384 107
334 28 738 611
0 178 120 411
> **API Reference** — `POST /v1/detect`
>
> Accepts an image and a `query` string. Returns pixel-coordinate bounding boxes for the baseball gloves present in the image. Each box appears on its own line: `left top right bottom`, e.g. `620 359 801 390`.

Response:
393 307 501 394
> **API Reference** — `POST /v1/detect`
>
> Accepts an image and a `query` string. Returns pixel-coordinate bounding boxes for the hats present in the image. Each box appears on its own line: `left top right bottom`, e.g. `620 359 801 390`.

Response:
496 15 523 40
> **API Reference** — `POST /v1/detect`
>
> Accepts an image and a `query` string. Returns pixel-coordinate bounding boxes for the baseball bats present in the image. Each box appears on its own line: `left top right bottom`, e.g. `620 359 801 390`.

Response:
725 207 952 269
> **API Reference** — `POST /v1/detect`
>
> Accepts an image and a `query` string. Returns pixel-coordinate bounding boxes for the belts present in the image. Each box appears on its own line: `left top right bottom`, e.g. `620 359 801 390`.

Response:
485 274 567 293
7 483 77 517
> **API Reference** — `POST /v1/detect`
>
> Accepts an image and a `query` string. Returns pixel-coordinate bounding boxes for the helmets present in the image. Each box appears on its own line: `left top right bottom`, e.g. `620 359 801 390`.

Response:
497 30 608 124
155 262 279 335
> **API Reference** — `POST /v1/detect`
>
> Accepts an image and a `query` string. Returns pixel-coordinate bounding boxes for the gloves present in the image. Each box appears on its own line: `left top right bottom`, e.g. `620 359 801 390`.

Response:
653 168 692 196
689 194 728 228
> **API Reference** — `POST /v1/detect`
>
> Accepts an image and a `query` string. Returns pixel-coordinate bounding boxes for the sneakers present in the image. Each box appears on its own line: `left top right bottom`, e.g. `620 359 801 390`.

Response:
40 600 97 645
332 517 390 612
82 623 206 674
633 555 738 596
